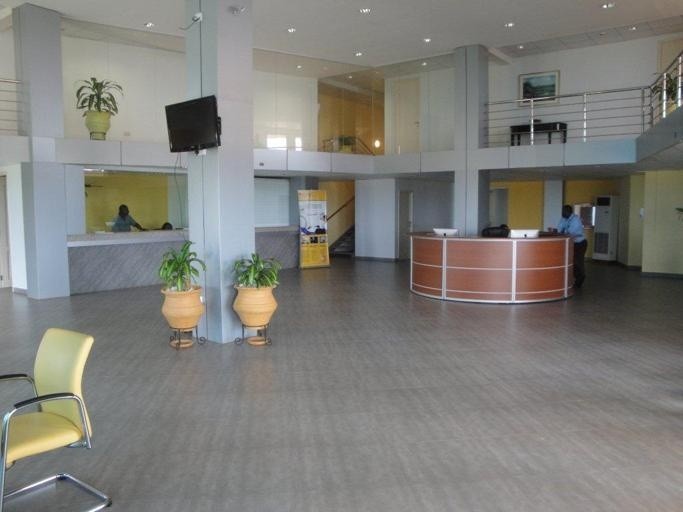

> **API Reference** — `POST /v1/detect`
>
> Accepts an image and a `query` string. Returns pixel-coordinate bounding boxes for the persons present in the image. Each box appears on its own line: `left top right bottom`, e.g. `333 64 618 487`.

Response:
547 205 588 289
112 204 149 232
162 222 173 230
500 223 511 238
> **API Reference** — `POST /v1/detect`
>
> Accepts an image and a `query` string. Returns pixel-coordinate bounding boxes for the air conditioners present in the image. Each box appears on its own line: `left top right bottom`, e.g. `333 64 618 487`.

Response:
591 195 619 262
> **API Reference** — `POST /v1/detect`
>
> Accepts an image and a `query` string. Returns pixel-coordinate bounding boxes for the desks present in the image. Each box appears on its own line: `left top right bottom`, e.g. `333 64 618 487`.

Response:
509 122 567 146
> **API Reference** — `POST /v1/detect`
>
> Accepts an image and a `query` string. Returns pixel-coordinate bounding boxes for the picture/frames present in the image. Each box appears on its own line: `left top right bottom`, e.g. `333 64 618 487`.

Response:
518 70 560 106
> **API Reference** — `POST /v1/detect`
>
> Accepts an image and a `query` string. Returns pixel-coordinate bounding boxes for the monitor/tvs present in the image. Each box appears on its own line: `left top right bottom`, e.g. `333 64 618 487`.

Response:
510 228 540 239
164 94 221 153
432 228 459 238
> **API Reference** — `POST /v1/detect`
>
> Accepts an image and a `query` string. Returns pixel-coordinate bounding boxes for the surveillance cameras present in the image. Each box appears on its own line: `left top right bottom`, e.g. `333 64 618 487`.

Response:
191 12 203 23
233 5 247 17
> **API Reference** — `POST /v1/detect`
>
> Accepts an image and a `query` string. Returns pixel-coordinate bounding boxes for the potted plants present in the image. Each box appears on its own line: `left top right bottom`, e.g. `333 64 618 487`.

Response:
651 73 678 119
158 242 206 350
76 77 124 140
232 254 283 346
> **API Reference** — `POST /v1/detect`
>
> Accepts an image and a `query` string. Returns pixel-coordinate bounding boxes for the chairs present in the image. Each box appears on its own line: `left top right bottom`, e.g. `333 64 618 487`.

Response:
482 227 508 237
0 328 111 512
